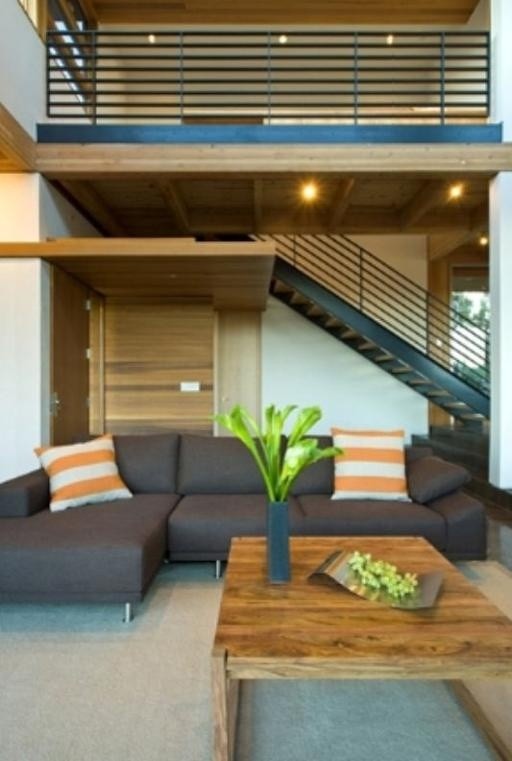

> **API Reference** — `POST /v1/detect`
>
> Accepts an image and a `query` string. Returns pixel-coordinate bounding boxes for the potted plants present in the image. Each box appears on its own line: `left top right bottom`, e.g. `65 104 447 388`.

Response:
208 401 344 584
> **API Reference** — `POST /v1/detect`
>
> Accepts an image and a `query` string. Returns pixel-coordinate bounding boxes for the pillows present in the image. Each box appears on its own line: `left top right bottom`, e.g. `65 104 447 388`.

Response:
287 435 333 495
34 433 134 512
176 432 268 495
72 434 178 494
405 454 472 503
329 427 413 502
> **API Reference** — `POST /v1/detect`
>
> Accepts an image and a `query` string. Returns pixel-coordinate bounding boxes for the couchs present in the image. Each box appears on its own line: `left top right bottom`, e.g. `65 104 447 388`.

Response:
1 435 490 623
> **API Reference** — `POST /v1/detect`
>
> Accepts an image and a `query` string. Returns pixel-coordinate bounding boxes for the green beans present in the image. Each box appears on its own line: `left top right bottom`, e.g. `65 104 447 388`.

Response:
348 549 419 600
